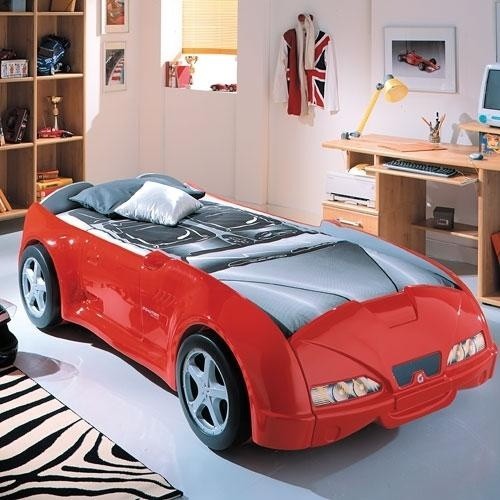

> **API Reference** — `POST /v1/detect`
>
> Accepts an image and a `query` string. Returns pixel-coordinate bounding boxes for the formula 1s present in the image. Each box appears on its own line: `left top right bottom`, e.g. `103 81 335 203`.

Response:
397 41 441 73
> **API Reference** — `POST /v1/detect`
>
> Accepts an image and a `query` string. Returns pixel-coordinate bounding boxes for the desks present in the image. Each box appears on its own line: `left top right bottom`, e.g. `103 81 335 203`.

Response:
321 133 500 309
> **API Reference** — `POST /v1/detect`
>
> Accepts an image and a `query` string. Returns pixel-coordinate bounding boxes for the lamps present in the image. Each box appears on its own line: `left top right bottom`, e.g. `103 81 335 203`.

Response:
340 74 408 139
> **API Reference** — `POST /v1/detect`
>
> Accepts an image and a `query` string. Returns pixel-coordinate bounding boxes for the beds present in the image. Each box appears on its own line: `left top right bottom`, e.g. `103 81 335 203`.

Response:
19 170 498 451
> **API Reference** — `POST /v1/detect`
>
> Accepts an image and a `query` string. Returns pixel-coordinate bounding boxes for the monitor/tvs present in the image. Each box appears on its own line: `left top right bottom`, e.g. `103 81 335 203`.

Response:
478 64 500 128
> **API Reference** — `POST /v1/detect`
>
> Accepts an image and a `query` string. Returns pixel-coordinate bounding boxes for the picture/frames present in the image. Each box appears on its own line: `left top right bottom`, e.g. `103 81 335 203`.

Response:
99 0 130 93
382 25 458 94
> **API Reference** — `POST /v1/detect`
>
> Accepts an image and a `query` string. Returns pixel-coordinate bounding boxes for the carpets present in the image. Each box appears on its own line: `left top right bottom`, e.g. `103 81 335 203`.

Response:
0 364 183 500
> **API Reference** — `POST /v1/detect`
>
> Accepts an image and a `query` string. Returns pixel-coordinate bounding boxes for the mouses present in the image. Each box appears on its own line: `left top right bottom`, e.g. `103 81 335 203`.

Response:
469 153 484 160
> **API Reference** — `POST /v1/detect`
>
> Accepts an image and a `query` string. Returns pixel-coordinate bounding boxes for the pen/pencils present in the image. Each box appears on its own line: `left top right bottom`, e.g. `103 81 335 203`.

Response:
421 112 446 130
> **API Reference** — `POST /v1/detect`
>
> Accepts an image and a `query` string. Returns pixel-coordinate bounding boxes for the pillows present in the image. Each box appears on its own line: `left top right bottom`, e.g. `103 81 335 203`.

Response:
67 174 208 227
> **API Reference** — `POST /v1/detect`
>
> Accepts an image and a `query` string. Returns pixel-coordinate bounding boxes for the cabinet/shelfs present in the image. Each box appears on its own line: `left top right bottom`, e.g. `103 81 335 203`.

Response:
0 0 87 235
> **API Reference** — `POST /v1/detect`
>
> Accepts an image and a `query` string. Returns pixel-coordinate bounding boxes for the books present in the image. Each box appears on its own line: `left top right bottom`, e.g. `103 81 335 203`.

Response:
37 167 73 201
0 190 16 212
166 60 191 88
50 0 76 12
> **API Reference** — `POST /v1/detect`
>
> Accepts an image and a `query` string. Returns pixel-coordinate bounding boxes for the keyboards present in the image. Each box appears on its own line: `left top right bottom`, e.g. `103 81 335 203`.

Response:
384 159 457 179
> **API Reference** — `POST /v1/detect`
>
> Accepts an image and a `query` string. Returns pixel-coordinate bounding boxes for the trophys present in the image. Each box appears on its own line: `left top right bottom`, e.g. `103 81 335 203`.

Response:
46 95 64 129
185 56 198 85
